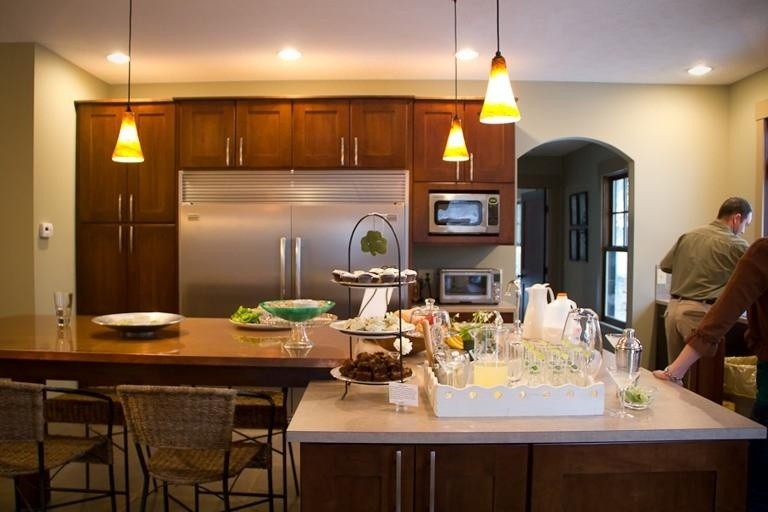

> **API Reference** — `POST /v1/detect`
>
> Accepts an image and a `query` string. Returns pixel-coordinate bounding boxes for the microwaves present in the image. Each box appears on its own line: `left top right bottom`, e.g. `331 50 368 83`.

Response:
438 267 501 305
427 191 500 235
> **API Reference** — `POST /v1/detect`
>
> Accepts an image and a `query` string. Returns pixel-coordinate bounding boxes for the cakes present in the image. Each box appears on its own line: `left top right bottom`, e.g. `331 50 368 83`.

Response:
344 314 402 333
340 352 412 381
331 267 417 284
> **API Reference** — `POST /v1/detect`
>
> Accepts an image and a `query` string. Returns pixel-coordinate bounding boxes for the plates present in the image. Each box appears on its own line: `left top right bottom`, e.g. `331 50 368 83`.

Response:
331 277 416 289
228 312 337 330
90 311 185 337
329 366 416 385
331 318 416 339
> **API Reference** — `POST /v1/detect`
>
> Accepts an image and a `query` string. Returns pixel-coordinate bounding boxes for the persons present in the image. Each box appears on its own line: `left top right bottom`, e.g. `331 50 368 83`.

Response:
660 196 753 401
651 235 768 512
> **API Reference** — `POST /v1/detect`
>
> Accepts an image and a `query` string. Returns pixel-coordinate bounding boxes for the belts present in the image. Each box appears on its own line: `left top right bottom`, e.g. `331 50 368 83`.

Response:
671 294 715 305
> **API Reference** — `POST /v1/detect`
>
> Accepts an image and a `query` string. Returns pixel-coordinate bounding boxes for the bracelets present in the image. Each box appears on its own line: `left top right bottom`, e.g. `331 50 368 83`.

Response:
663 366 683 382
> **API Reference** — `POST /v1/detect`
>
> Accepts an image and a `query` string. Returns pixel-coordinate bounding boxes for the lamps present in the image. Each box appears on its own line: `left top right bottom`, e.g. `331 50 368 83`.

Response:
441 0 521 163
109 1 146 166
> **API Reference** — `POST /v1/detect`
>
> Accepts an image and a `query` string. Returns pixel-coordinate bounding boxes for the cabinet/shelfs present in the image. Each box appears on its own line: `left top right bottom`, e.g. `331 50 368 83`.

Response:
74 97 176 314
414 95 517 183
300 444 527 509
293 94 414 171
175 97 292 170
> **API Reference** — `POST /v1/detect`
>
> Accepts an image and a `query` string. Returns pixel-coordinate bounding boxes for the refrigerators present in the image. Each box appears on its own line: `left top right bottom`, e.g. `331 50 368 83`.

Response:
176 168 409 322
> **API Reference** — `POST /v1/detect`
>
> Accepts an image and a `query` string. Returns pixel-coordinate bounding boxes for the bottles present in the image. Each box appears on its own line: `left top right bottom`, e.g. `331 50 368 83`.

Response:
611 328 643 399
421 299 438 324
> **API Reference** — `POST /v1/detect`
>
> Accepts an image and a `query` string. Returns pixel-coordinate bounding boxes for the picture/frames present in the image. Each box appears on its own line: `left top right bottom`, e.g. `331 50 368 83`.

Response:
569 191 589 262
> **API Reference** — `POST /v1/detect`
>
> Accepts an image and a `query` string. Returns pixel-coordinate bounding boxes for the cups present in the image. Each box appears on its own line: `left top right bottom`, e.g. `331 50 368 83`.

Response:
429 307 601 389
604 332 622 349
624 385 659 411
52 291 72 328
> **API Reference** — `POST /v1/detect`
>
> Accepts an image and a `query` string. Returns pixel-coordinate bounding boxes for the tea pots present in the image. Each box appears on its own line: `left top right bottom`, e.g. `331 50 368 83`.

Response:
522 282 555 342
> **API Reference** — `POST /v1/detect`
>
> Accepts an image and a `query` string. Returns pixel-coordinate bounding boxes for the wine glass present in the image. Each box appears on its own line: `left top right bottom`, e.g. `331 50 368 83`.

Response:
606 366 640 419
260 299 336 349
